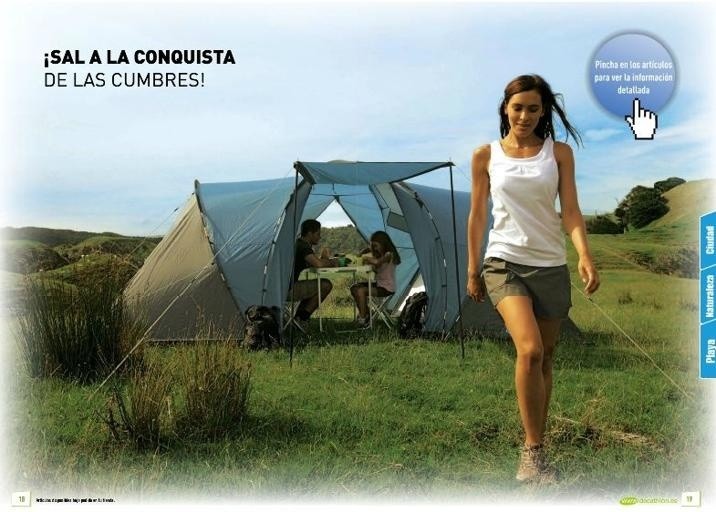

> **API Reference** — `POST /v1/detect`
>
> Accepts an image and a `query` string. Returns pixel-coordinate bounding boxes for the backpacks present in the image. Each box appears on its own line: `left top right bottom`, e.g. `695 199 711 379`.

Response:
395 291 428 338
240 303 282 351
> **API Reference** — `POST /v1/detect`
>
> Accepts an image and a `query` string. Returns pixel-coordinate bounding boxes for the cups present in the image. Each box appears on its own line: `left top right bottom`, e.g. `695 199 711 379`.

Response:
337 258 346 267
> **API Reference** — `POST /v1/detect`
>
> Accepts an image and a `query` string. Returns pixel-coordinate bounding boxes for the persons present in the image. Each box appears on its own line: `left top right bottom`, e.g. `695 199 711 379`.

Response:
463 74 602 486
350 230 401 327
285 219 335 337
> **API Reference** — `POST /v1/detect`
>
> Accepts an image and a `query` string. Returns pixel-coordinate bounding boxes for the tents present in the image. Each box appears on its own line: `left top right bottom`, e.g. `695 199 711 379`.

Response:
112 160 590 345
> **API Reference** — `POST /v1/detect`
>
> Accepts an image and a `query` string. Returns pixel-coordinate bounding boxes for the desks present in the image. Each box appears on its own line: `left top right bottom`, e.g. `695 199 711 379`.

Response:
303 265 374 334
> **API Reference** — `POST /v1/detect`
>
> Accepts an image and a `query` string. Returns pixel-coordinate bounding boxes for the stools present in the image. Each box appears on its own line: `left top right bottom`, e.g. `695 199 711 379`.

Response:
366 292 396 329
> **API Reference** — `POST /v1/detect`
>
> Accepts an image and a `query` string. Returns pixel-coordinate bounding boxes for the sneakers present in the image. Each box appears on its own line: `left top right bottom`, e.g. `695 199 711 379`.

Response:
515 444 544 482
291 317 308 335
352 309 373 327
